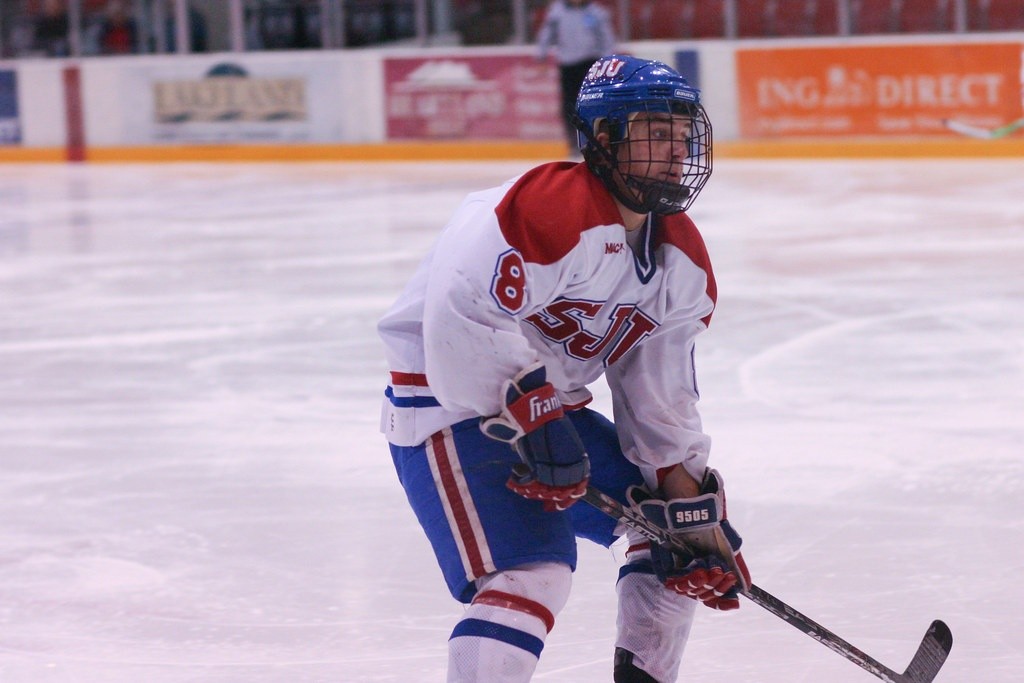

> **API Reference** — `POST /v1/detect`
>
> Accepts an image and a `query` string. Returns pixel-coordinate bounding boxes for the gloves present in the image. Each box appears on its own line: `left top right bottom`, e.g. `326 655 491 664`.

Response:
625 468 751 610
479 369 591 513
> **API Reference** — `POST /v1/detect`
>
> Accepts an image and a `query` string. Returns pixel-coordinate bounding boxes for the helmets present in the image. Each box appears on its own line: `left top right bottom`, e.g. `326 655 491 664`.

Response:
570 56 713 214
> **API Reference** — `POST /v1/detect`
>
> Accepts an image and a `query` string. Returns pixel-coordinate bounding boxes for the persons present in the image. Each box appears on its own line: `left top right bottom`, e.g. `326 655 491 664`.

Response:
376 54 751 683
537 0 616 157
28 0 208 55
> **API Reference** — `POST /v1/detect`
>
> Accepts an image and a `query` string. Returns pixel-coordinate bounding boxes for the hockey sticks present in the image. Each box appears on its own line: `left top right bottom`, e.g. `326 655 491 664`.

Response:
509 461 955 683
